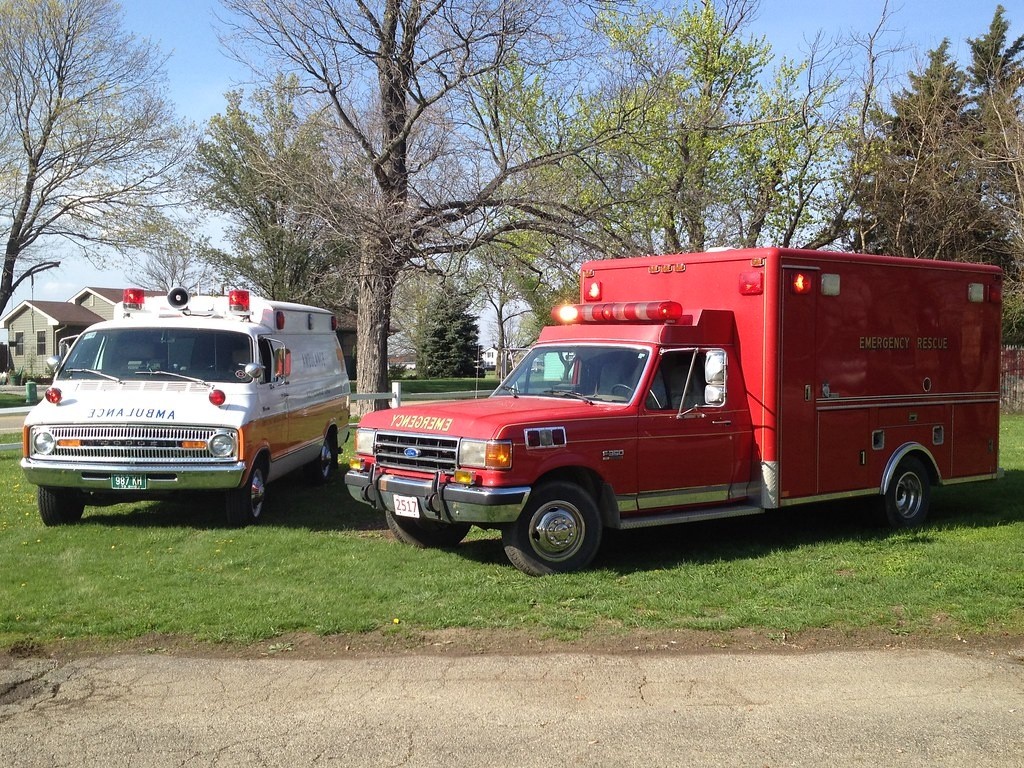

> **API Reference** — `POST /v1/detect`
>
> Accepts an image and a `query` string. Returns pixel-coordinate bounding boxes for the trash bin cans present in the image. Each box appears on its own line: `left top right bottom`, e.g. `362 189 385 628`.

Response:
24 382 38 403
11 374 21 386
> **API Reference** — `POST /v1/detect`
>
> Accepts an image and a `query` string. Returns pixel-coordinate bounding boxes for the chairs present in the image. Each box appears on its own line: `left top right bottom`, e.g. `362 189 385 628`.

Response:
595 362 628 399
665 364 704 410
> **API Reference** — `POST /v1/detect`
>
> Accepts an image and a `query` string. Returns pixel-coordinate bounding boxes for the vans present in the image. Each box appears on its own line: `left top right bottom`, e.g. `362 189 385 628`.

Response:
19 286 351 528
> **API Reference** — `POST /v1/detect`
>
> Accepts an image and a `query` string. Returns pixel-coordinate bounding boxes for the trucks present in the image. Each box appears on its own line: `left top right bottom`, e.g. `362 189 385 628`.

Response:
343 246 1005 577
